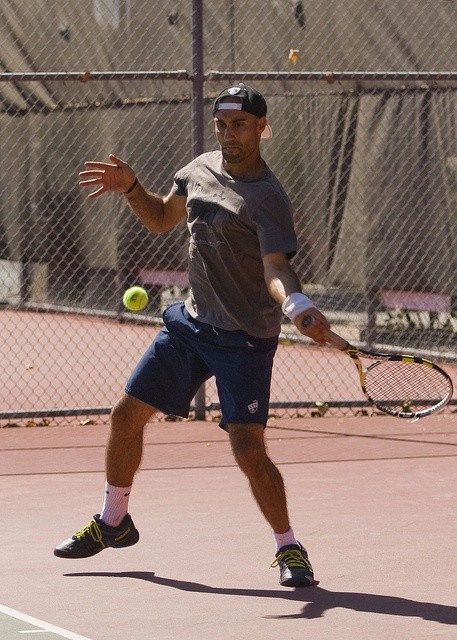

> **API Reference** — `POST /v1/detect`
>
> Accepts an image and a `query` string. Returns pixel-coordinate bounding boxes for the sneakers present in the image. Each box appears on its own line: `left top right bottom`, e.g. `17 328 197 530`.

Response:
52 513 139 559
268 539 314 586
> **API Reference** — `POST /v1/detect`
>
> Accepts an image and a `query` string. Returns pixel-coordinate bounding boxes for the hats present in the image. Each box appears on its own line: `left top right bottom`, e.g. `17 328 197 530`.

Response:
212 85 271 141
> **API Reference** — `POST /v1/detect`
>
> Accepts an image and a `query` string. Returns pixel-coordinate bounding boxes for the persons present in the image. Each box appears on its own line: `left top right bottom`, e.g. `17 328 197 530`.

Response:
53 81 330 586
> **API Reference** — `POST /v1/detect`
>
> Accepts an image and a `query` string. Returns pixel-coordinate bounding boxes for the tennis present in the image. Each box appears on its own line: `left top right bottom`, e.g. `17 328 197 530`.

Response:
122 286 149 311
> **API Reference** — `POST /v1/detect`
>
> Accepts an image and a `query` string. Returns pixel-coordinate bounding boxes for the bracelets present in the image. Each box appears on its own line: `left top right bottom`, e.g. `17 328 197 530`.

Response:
124 177 138 193
282 292 317 324
